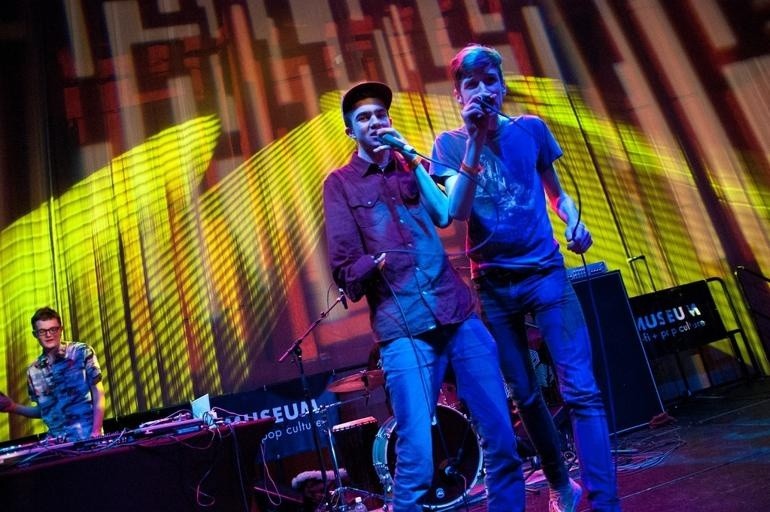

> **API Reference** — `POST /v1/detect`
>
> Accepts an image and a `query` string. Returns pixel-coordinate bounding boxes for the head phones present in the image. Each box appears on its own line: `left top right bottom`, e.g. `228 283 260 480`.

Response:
38 341 68 368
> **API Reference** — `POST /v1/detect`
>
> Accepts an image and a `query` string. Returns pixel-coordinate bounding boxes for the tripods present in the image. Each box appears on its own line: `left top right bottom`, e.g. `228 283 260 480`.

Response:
279 298 393 511
523 424 544 482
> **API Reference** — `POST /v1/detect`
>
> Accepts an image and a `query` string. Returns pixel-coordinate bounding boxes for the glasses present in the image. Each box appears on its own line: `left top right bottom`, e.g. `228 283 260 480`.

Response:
34 326 61 336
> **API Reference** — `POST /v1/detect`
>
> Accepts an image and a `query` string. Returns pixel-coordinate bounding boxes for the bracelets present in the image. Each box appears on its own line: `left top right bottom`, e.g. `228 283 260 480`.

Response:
409 157 425 171
459 162 483 174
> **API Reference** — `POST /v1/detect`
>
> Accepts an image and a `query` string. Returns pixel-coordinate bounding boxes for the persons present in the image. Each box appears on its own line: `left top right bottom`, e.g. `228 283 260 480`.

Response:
1 304 107 441
430 45 626 511
322 80 527 511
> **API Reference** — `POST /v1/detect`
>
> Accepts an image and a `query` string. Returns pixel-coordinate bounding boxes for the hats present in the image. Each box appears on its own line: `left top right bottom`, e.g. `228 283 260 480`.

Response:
338 78 398 116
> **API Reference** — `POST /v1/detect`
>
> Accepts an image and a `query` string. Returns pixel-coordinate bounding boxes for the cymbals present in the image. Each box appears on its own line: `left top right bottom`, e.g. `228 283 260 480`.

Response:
327 370 384 392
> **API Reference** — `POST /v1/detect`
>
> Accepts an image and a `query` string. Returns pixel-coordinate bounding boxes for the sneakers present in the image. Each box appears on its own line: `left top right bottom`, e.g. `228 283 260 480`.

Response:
544 477 584 512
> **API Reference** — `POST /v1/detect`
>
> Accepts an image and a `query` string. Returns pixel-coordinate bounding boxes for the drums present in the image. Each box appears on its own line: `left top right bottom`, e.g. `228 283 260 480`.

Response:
332 416 385 512
372 403 483 511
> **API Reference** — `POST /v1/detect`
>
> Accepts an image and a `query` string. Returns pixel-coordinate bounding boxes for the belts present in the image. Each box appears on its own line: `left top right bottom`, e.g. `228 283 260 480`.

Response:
475 267 554 290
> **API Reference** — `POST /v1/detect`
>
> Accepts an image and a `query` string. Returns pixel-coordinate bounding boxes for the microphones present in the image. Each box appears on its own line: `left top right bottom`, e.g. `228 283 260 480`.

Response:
339 287 349 310
376 133 417 154
475 91 492 101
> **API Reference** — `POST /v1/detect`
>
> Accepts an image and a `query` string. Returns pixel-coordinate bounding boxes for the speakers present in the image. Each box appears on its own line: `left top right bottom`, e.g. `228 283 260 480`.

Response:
573 270 665 437
